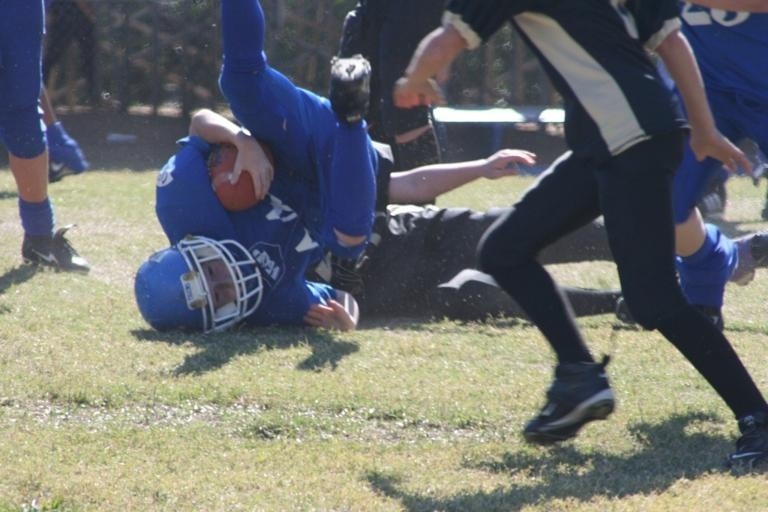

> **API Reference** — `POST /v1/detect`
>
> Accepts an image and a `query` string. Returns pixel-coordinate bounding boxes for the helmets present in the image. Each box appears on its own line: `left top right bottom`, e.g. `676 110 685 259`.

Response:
136 234 264 336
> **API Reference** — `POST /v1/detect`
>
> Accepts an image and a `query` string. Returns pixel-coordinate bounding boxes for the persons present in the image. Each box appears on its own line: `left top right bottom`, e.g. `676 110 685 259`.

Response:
0 0 95 279
129 105 766 336
207 2 536 331
327 2 446 210
640 1 765 338
392 1 766 478
36 76 90 185
699 183 726 210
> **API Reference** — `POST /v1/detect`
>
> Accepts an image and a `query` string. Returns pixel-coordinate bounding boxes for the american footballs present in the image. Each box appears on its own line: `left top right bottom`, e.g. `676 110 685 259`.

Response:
208 140 273 210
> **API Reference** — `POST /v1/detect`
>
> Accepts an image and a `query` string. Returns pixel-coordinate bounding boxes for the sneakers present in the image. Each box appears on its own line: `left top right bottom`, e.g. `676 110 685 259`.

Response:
524 355 614 441
22 226 90 273
332 258 368 317
330 54 372 118
730 414 768 460
49 123 87 183
